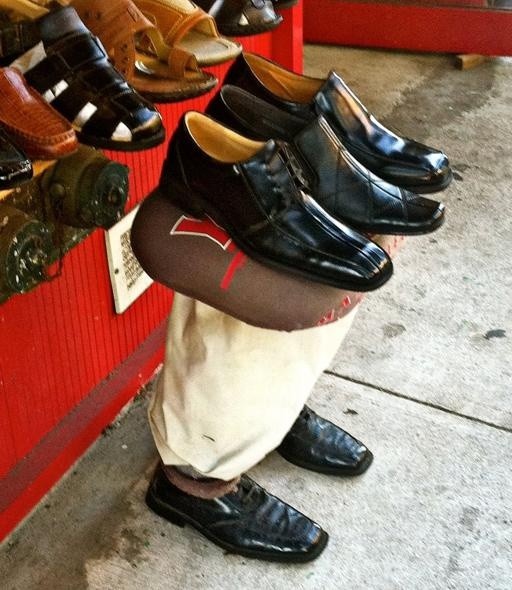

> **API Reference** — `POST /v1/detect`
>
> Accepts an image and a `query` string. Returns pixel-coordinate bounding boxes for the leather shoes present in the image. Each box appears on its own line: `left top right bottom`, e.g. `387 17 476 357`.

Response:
277 405 373 476
145 461 328 562
